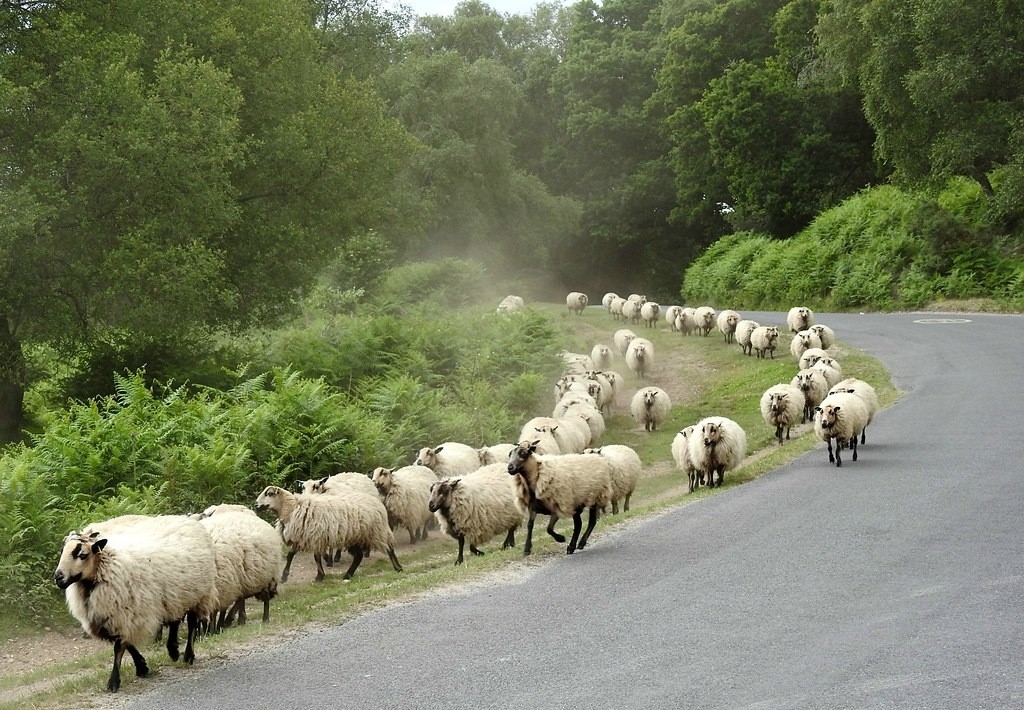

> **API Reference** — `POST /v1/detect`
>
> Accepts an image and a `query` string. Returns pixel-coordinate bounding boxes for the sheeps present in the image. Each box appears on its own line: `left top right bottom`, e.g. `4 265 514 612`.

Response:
55 288 877 695
